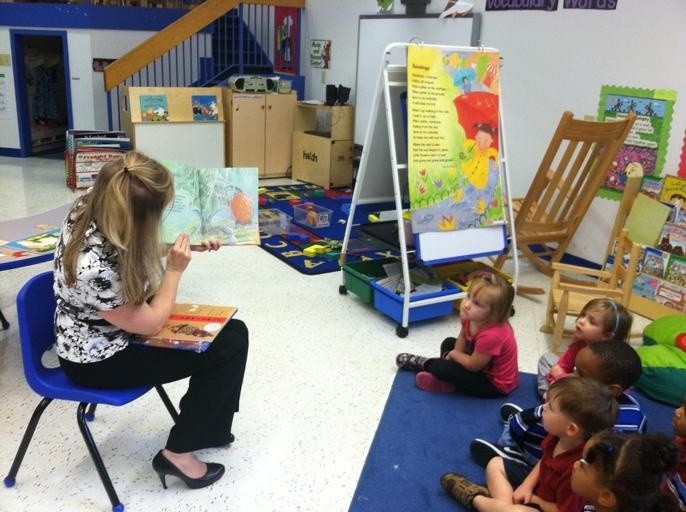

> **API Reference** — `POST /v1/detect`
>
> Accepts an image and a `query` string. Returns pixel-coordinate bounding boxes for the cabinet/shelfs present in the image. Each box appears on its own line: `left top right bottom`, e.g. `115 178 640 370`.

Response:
224 90 299 178
290 101 354 192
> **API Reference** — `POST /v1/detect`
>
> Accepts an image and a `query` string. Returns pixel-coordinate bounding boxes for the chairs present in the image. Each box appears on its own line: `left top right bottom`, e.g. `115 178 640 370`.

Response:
539 227 645 360
484 110 640 298
3 271 180 512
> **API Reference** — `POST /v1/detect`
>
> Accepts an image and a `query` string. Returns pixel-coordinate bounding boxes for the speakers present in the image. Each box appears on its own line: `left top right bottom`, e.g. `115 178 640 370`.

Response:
337 84 351 105
324 85 338 105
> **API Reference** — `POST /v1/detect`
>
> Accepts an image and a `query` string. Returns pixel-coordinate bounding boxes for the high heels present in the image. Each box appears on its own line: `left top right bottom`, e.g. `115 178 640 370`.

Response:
152 448 225 491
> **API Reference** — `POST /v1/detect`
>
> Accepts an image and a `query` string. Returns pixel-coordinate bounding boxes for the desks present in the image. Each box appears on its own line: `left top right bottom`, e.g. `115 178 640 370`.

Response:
1 204 76 330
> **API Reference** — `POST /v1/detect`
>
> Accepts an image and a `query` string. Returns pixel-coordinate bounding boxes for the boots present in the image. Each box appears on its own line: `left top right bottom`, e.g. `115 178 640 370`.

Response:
415 370 456 394
395 352 429 372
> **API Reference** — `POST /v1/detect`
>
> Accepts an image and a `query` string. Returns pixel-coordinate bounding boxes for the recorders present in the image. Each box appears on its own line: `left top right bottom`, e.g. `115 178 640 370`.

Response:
228 74 276 94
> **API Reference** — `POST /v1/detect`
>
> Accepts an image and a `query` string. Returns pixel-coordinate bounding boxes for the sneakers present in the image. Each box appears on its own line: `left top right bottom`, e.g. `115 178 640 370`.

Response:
468 437 528 469
499 401 524 421
439 472 490 512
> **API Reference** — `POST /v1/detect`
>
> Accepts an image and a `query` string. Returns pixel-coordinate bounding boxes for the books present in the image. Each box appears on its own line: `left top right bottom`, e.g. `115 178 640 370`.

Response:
3 226 63 256
139 94 170 123
128 302 238 354
190 94 220 124
149 159 263 247
599 166 686 310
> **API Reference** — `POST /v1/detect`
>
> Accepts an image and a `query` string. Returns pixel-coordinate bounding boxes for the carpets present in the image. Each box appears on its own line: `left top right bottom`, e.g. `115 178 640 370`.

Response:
348 365 678 511
257 177 410 276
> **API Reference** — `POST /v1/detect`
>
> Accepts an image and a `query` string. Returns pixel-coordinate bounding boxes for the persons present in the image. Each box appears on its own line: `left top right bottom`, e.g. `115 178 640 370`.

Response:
442 296 685 512
51 150 249 491
396 270 521 398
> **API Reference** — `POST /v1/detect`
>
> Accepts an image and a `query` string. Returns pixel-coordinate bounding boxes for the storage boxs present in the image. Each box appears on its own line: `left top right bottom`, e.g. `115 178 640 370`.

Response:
369 274 462 326
432 261 514 313
258 208 293 236
344 256 403 304
292 203 334 229
